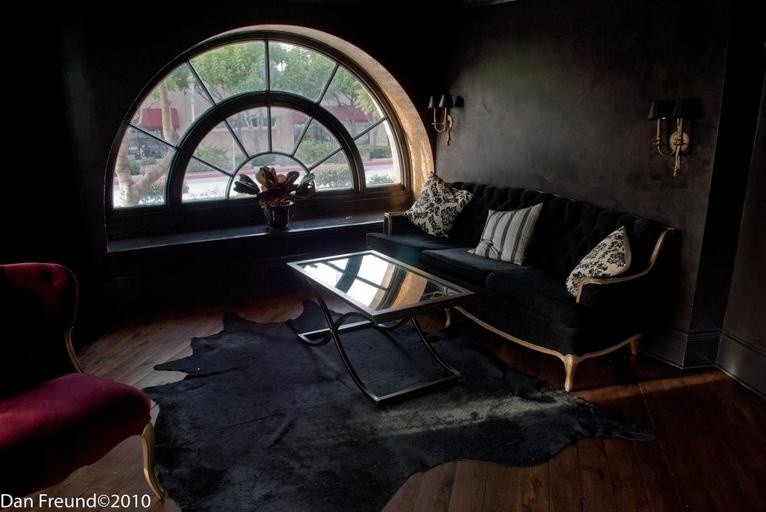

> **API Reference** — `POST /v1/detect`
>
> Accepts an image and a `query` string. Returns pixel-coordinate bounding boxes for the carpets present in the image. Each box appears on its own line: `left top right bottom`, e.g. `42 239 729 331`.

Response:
139 299 658 512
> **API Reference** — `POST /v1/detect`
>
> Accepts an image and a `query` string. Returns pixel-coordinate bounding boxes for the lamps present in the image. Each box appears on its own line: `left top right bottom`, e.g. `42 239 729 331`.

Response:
647 97 703 178
427 93 455 146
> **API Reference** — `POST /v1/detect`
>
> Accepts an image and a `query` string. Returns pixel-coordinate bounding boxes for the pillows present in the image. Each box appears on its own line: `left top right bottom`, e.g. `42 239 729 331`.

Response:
465 201 545 266
566 225 632 297
404 171 474 238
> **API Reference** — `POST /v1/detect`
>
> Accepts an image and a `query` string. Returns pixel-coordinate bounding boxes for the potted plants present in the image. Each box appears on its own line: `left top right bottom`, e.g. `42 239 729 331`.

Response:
233 166 316 229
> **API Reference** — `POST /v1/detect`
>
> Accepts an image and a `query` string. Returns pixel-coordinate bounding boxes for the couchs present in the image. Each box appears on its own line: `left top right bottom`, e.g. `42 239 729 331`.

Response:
0 264 165 507
367 181 684 393
